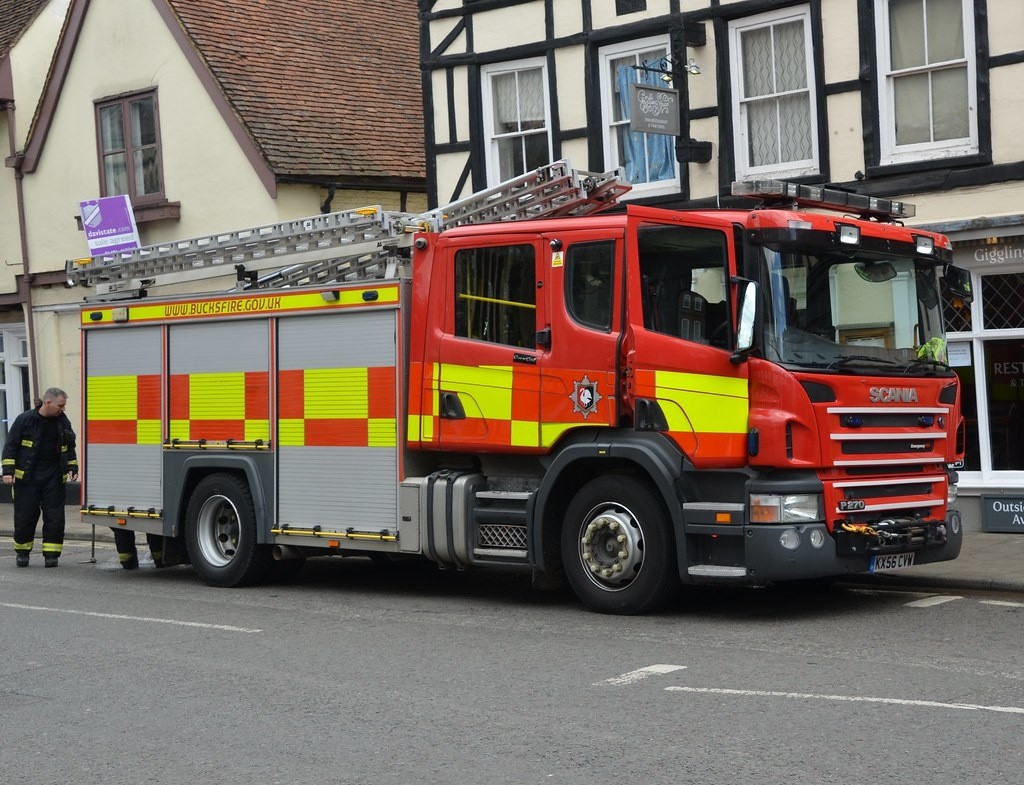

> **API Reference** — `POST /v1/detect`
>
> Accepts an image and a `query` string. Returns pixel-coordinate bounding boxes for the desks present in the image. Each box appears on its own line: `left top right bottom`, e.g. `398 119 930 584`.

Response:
957 416 1011 471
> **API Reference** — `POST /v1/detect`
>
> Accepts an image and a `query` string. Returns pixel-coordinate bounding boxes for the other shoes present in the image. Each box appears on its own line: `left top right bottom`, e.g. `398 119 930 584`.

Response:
123 558 139 570
45 557 58 567
16 553 29 567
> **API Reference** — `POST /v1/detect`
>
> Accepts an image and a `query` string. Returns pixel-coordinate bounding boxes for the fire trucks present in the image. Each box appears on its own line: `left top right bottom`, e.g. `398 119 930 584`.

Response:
79 158 975 617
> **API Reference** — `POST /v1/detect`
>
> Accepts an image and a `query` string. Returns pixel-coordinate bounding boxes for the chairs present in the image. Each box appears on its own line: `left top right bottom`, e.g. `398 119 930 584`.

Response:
647 276 684 334
766 273 792 326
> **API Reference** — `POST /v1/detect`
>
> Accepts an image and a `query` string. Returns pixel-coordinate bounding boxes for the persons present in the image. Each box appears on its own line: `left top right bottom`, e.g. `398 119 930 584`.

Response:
1 387 75 567
110 527 165 572
